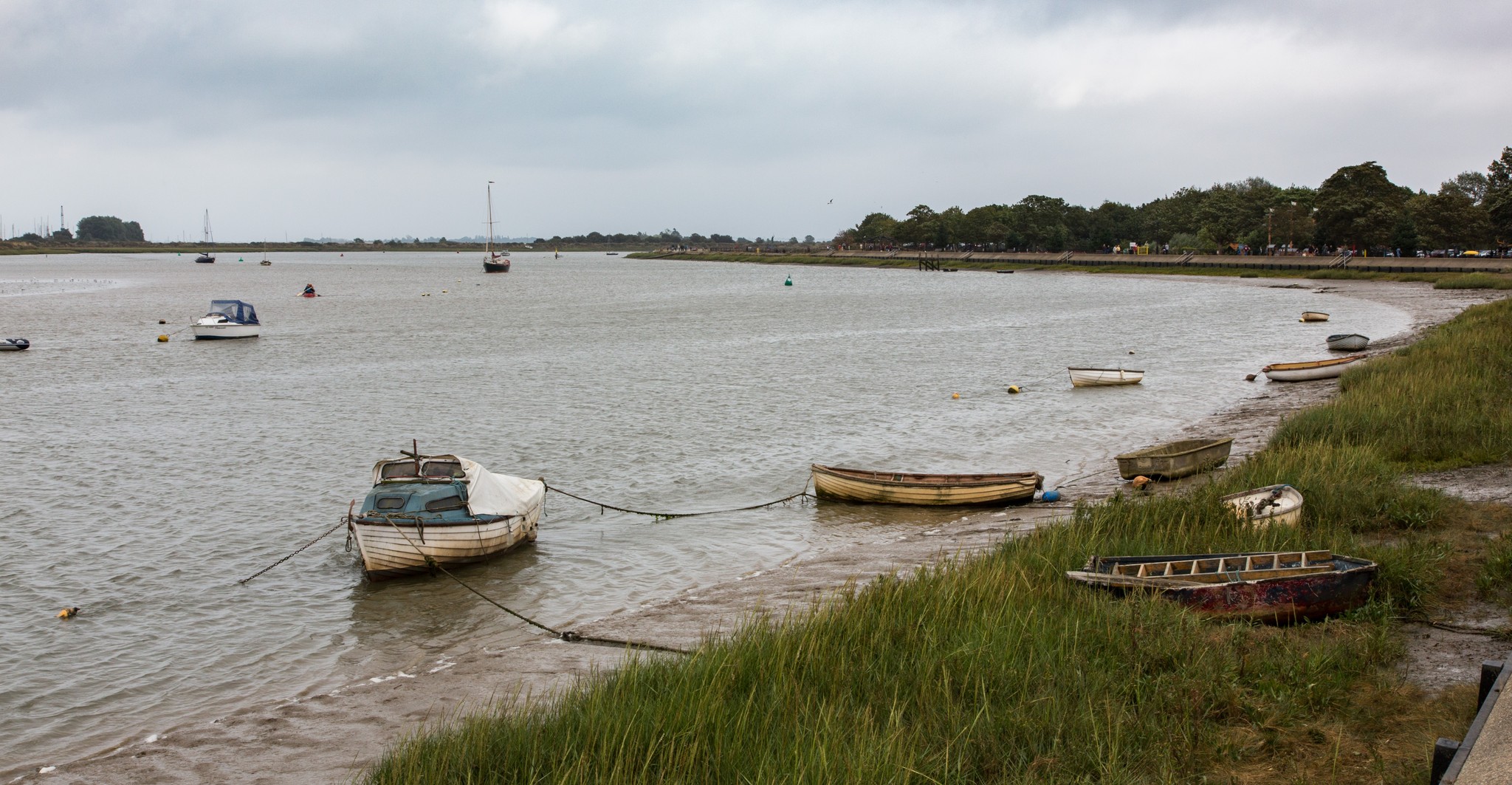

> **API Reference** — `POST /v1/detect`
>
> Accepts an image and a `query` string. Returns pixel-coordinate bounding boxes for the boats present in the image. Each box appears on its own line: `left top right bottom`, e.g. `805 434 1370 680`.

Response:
1218 484 1303 531
943 268 959 272
1114 435 1236 482
996 270 1014 274
692 248 697 251
524 245 533 249
1264 353 1370 382
810 462 1040 508
1065 365 1145 395
1064 552 1380 617
1315 333 1370 351
190 300 261 343
346 438 547 583
0 338 30 351
1299 311 1330 324
303 293 317 298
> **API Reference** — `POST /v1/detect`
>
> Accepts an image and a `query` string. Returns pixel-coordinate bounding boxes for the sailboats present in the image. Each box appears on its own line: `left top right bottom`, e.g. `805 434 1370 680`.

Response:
480 181 510 275
195 209 216 264
260 237 271 266
606 232 618 255
